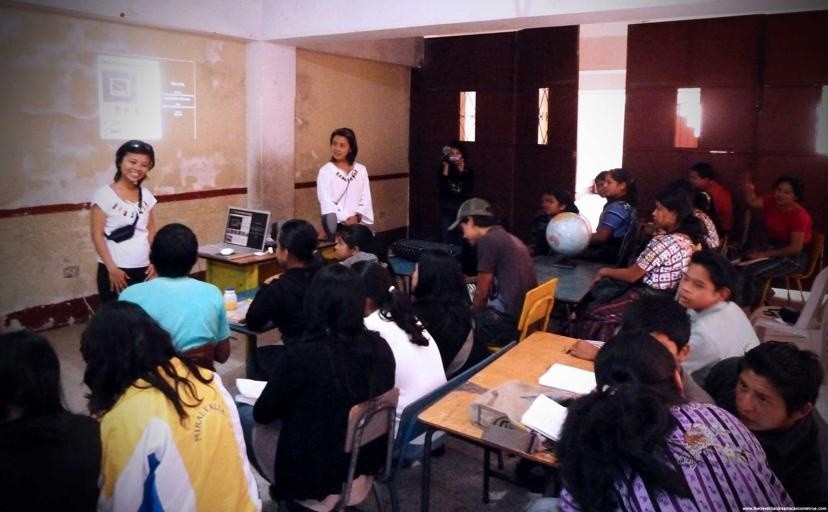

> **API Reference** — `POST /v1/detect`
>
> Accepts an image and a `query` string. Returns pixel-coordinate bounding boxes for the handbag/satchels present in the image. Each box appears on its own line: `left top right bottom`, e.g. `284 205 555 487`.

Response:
109 225 134 242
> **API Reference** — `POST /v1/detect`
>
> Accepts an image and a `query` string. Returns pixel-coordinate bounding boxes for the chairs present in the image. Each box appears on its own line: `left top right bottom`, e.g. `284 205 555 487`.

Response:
488 277 558 355
393 341 516 511
295 385 400 512
755 229 826 307
749 268 828 382
178 342 214 372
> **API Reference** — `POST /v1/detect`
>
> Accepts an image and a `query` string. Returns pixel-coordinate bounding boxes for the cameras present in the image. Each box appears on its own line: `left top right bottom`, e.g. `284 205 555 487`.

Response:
441 151 460 163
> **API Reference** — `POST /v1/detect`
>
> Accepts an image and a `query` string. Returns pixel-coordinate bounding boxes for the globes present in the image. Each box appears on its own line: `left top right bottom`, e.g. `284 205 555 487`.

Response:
545 212 592 270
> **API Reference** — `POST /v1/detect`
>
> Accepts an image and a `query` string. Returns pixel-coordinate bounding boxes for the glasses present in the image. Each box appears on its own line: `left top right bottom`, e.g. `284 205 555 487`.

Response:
129 141 152 150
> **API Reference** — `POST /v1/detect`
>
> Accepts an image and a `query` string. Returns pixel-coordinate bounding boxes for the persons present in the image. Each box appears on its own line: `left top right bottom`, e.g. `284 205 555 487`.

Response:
78 300 263 512
239 263 397 511
117 221 231 369
2 330 100 509
89 139 159 303
348 161 827 511
245 217 336 380
438 137 475 244
314 126 374 234
334 222 381 272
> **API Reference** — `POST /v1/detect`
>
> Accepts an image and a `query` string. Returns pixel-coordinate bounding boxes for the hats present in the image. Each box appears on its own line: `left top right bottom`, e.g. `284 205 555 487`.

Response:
448 197 492 231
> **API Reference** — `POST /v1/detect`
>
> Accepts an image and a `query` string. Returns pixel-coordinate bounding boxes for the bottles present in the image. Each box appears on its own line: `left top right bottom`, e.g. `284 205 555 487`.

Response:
223 288 238 321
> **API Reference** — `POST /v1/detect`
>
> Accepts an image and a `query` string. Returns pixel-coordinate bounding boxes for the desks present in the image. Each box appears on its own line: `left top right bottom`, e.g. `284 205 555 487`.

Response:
530 251 616 317
388 244 463 300
223 258 419 376
417 329 604 512
197 233 338 294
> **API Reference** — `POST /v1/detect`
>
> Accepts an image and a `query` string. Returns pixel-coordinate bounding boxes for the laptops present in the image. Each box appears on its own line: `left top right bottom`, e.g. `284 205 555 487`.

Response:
195 206 272 259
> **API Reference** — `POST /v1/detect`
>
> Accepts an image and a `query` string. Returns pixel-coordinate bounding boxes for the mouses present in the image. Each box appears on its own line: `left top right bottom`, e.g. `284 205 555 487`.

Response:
220 248 234 256
